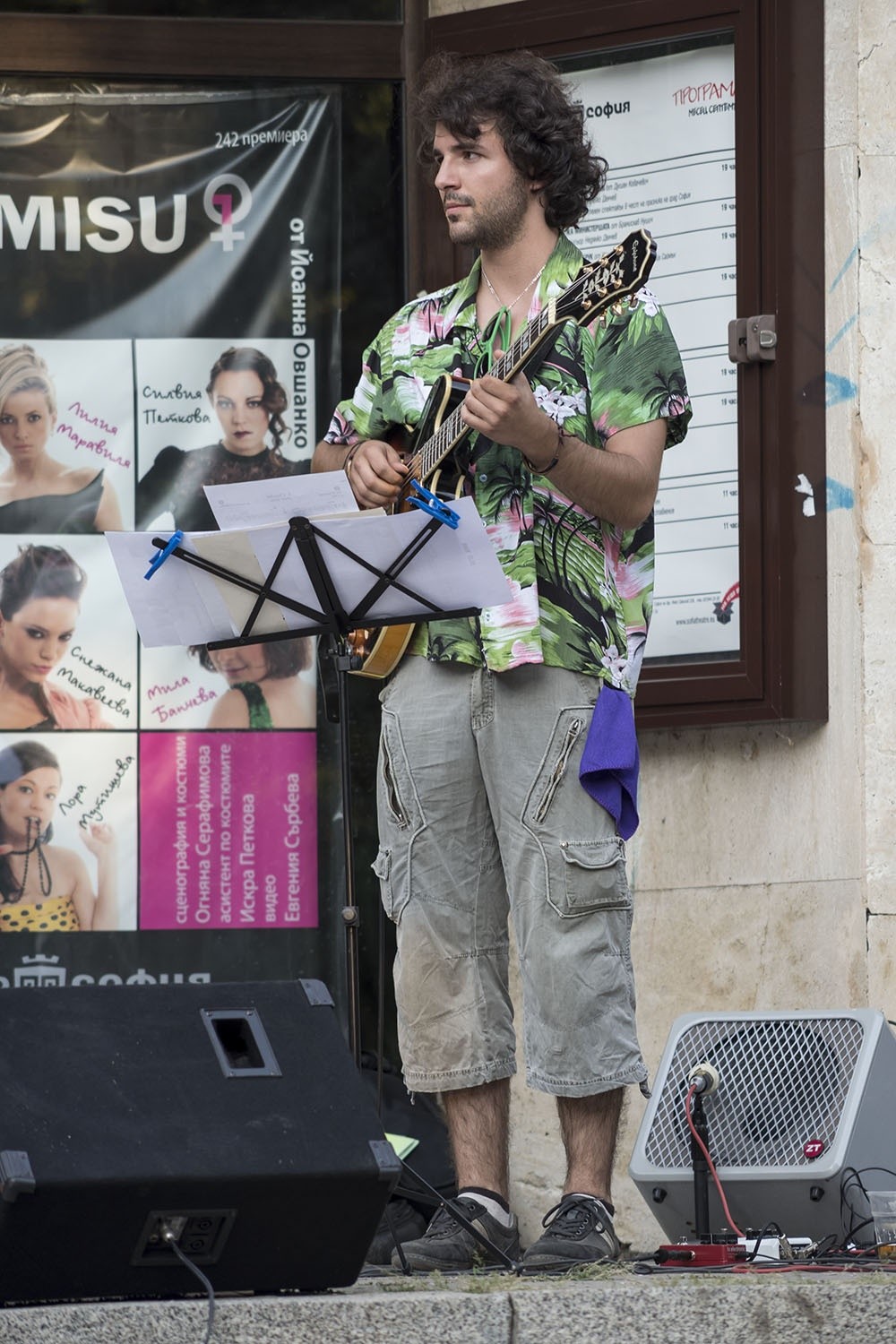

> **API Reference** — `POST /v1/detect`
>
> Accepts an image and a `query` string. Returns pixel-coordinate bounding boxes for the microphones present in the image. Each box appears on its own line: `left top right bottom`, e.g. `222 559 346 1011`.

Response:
686 1064 720 1097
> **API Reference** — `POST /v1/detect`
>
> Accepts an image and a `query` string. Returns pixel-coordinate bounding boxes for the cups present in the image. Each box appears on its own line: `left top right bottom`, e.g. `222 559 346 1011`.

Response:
866 1190 896 1264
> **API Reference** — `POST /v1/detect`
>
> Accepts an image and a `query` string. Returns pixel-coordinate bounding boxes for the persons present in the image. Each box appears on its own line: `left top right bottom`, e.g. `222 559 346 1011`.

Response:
188 637 317 728
137 348 311 532
311 52 698 1286
0 741 120 933
0 342 123 533
0 542 116 730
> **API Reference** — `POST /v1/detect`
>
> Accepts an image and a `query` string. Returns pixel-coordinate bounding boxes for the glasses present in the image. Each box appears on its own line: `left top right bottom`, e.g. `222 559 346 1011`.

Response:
474 305 512 378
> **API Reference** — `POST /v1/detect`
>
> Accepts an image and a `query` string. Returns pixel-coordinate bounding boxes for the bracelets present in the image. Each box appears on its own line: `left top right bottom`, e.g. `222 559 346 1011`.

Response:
340 440 367 479
521 423 578 474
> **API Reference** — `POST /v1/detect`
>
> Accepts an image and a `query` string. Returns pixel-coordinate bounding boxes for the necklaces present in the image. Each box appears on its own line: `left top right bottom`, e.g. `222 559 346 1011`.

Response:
4 817 52 903
482 265 546 311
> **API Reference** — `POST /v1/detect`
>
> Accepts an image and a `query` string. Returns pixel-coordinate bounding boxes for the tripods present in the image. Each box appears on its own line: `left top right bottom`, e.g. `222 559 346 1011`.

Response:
132 496 525 1267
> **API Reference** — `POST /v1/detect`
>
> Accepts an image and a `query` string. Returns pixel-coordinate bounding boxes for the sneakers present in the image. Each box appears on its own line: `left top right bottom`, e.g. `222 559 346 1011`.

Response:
515 1192 630 1270
391 1196 520 1267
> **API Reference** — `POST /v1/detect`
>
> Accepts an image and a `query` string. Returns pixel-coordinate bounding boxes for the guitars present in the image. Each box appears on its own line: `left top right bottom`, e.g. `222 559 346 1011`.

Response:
336 228 658 680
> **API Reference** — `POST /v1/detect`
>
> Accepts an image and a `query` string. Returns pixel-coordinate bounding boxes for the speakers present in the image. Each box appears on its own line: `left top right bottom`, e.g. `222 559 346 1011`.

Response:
625 1013 896 1247
1 979 403 1308
